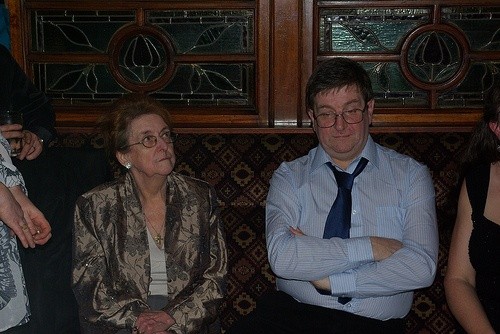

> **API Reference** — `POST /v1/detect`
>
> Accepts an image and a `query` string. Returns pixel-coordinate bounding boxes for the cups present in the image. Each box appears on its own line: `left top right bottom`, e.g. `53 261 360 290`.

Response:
0 110 25 157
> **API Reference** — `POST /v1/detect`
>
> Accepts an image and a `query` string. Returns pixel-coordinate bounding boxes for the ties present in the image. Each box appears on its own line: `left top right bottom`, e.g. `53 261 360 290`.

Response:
322 157 369 306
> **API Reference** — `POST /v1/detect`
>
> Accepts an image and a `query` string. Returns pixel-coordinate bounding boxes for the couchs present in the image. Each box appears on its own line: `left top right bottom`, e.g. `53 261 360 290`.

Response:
56 132 462 334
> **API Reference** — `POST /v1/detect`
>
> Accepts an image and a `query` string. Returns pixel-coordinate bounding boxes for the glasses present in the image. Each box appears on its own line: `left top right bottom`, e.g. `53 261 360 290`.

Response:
123 131 173 149
312 101 368 128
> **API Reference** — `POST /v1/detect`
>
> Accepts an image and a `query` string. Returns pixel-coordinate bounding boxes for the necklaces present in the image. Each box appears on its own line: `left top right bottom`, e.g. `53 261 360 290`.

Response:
146 215 165 245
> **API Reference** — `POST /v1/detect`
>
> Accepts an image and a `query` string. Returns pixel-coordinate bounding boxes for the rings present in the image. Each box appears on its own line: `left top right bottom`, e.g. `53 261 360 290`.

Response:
36 230 41 233
137 328 144 334
133 326 138 332
20 223 28 230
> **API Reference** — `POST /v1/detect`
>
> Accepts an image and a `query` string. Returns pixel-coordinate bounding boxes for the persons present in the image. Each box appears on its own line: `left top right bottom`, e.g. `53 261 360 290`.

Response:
443 77 500 334
71 94 228 334
0 42 56 161
0 132 52 334
224 57 439 334
